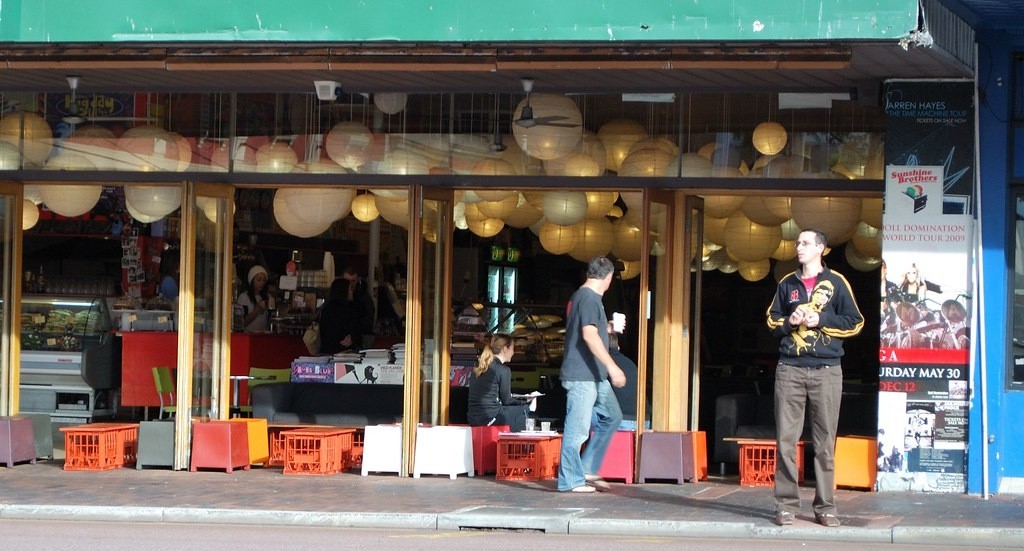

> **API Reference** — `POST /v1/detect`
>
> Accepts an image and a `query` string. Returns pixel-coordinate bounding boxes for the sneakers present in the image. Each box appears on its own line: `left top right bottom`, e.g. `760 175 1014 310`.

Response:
817 514 840 526
776 510 795 525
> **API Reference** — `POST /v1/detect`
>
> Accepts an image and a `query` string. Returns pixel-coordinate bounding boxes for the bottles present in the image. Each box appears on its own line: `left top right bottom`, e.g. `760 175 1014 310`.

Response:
23 265 46 293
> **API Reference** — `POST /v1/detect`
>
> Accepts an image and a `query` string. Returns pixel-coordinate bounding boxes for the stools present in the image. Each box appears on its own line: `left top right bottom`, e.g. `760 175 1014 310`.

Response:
830 435 877 492
0 415 54 467
136 417 267 474
362 419 511 479
590 428 709 486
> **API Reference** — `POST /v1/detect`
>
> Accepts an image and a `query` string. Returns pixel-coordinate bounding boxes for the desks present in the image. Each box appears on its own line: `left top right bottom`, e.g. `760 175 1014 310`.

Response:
113 330 312 417
252 381 567 428
193 375 254 418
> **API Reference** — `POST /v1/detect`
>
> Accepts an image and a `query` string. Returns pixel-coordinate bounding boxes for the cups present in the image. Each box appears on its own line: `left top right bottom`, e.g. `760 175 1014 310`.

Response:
524 417 535 431
541 422 551 432
613 313 625 333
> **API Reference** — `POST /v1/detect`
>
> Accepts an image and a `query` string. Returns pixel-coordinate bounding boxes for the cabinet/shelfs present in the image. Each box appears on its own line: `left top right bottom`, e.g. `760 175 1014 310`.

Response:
19 293 118 459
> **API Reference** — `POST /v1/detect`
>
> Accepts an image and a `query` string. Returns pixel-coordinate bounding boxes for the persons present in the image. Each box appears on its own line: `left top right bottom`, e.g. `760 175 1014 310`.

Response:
468 333 535 431
607 334 636 420
238 265 272 331
881 259 943 322
557 259 627 492
159 250 179 305
318 267 375 354
766 228 864 527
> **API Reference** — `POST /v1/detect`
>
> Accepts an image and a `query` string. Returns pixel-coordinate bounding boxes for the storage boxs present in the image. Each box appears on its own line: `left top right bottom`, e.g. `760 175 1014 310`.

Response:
509 370 547 389
60 421 139 469
495 435 564 481
292 362 334 381
735 440 808 488
266 427 363 474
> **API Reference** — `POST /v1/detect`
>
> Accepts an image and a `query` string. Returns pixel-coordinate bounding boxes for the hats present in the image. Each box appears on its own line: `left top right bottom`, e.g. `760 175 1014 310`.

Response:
247 265 268 285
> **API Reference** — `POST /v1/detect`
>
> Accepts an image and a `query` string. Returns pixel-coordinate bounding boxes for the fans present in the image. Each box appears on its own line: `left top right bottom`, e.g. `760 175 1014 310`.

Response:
443 77 583 133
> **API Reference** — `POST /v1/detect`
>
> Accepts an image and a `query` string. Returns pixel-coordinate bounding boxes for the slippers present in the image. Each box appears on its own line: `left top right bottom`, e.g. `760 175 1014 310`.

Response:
585 474 612 491
560 484 596 493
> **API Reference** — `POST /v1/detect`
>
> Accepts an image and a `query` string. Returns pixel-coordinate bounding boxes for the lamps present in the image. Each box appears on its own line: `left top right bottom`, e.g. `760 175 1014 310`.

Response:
0 58 887 281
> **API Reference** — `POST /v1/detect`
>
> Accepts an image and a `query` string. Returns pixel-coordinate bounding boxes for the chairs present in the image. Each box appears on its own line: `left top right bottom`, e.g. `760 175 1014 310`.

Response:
150 366 291 422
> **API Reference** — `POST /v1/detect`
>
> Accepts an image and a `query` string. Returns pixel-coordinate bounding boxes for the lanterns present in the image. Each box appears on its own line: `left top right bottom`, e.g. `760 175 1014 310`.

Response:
0 90 884 281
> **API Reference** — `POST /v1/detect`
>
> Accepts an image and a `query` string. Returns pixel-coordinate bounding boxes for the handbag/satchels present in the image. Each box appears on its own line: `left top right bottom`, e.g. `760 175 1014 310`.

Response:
82 295 122 390
303 322 321 356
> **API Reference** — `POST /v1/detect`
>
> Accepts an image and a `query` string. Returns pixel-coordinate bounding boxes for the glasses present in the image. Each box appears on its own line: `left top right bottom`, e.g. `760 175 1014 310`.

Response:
794 241 818 247
